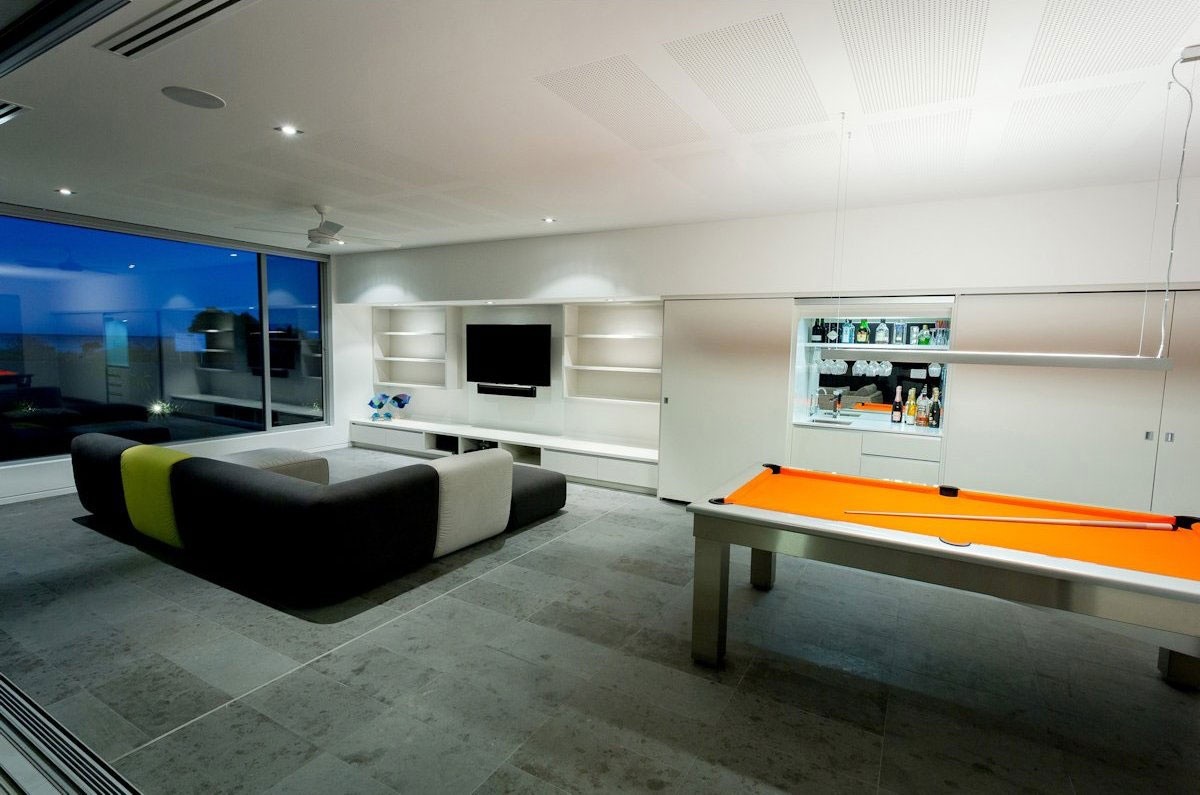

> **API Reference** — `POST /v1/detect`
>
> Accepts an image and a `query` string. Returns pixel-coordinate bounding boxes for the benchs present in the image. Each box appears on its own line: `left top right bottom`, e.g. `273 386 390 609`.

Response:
198 447 330 486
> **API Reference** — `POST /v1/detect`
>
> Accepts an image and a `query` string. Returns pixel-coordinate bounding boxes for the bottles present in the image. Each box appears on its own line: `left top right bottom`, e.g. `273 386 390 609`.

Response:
831 319 890 344
820 319 826 342
811 317 823 342
905 384 942 428
918 324 932 345
891 385 903 424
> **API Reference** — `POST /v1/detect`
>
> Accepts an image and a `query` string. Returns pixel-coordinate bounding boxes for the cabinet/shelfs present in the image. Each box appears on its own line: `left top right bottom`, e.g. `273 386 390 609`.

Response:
168 395 324 430
348 418 660 496
294 307 324 379
562 300 662 407
796 318 951 436
189 309 251 374
372 303 459 391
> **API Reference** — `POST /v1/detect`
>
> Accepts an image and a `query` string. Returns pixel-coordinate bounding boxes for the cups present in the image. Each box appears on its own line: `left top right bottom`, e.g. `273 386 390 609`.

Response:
928 362 942 377
893 319 907 344
814 359 890 377
910 326 919 345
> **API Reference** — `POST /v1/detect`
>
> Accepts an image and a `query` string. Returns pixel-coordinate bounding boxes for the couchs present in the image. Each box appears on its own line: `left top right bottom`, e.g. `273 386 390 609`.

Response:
71 431 514 611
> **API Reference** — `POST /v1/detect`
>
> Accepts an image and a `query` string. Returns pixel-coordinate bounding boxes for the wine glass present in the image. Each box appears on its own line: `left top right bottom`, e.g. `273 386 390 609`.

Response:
930 327 947 345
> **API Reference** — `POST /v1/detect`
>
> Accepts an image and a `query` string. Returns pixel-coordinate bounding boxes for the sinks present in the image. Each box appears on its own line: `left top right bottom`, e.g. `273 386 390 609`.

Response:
820 411 862 417
805 419 852 426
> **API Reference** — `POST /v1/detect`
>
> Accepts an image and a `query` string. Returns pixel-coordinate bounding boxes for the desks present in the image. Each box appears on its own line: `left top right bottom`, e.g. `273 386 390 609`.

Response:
685 462 1200 669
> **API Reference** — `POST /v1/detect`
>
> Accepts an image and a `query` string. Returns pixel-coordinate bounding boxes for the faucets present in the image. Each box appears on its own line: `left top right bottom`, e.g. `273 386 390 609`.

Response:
827 391 841 418
809 387 827 414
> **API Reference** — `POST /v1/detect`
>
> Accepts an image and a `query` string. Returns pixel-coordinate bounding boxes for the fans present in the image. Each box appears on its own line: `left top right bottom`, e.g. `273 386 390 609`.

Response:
230 206 401 253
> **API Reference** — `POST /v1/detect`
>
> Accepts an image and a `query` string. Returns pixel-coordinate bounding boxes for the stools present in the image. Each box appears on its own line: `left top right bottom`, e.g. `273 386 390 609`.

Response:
507 463 566 532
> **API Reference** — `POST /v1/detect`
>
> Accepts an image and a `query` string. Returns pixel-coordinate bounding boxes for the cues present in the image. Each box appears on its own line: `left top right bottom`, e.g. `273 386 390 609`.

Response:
845 510 1178 531
